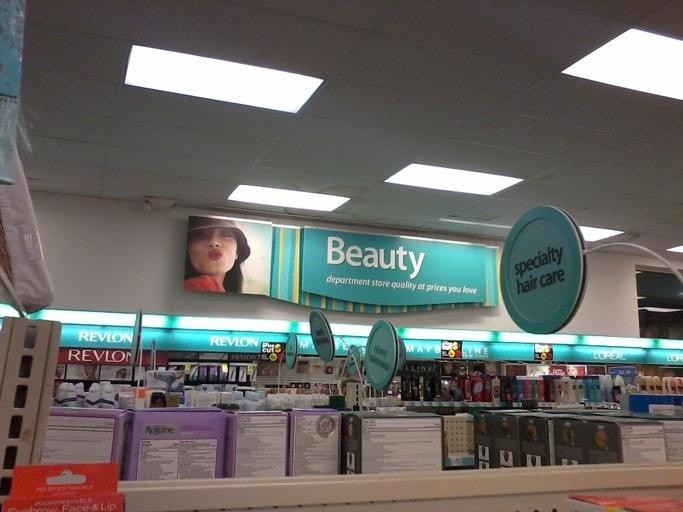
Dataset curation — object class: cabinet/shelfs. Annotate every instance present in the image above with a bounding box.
[120,467,543,512]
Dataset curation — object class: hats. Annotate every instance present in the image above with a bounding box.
[188,216,251,263]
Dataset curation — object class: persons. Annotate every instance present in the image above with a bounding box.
[151,392,169,411]
[186,217,250,296]
[55,362,65,379]
[82,363,99,382]
[442,363,454,374]
[486,361,498,377]
[114,367,127,380]
